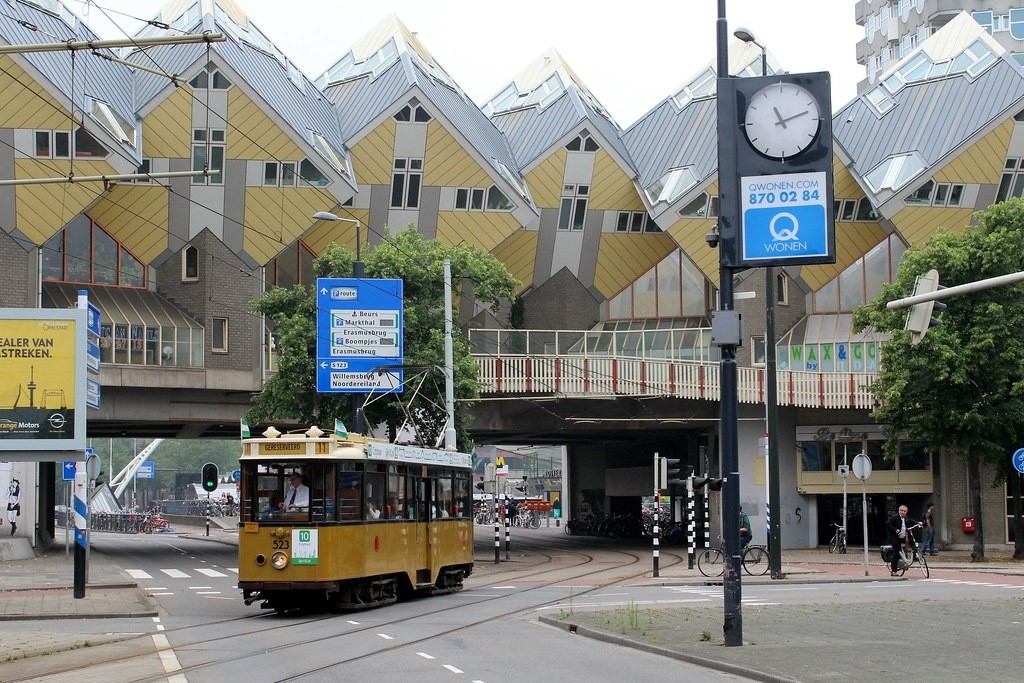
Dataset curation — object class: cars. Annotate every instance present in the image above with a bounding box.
[55,504,71,518]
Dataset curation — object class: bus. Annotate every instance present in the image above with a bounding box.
[238,426,475,618]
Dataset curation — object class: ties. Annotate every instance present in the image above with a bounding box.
[289,489,297,510]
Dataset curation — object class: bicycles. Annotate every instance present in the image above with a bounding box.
[210,500,240,517]
[880,522,929,578]
[828,523,847,555]
[139,518,169,533]
[476,507,541,529]
[564,512,689,543]
[697,536,771,576]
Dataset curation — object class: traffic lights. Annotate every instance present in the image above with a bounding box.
[667,458,680,488]
[478,483,484,492]
[205,466,215,489]
[710,478,722,491]
[929,285,949,330]
[95,471,104,488]
[693,477,711,488]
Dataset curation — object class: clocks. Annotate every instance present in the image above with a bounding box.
[741,81,825,164]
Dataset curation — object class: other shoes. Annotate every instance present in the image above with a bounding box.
[922,552,927,555]
[930,553,938,556]
[891,572,899,576]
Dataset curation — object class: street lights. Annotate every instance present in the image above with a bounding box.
[170,440,181,473]
[313,210,360,261]
[732,25,794,584]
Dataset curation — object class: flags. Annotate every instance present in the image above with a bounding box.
[241,418,250,438]
[335,418,348,439]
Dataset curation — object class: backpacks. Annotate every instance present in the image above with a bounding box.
[921,512,932,528]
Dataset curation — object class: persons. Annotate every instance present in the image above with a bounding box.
[380,496,449,519]
[278,472,310,512]
[739,504,752,565]
[498,498,523,526]
[219,492,234,517]
[887,505,923,576]
[921,502,937,556]
[365,497,380,520]
